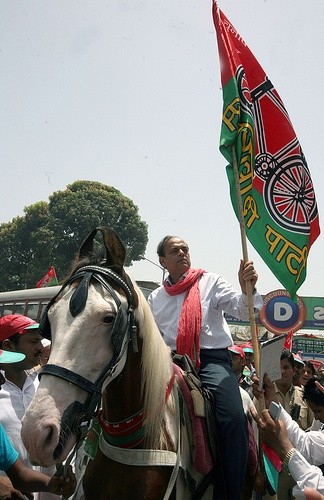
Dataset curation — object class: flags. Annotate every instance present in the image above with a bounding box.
[212,0,321,302]
[36,269,58,288]
[281,331,293,353]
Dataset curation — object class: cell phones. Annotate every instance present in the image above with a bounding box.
[268,401,281,421]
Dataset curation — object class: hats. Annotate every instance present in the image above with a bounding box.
[0,314,41,341]
[240,344,254,355]
[0,350,26,363]
[228,344,242,358]
[293,355,304,368]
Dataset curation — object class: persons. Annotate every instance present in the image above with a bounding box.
[227,341,324,500]
[146,236,245,500]
[0,313,78,500]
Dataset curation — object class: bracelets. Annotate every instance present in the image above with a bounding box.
[241,287,257,297]
[280,447,300,476]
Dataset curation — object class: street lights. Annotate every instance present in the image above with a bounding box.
[138,254,166,287]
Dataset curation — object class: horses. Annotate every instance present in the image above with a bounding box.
[22,227,260,500]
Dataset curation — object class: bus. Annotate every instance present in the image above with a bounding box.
[0,285,66,323]
